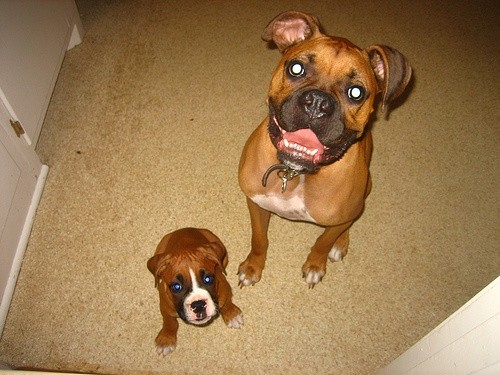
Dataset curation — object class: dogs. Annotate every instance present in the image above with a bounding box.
[236,9,412,291]
[147,227,246,357]
